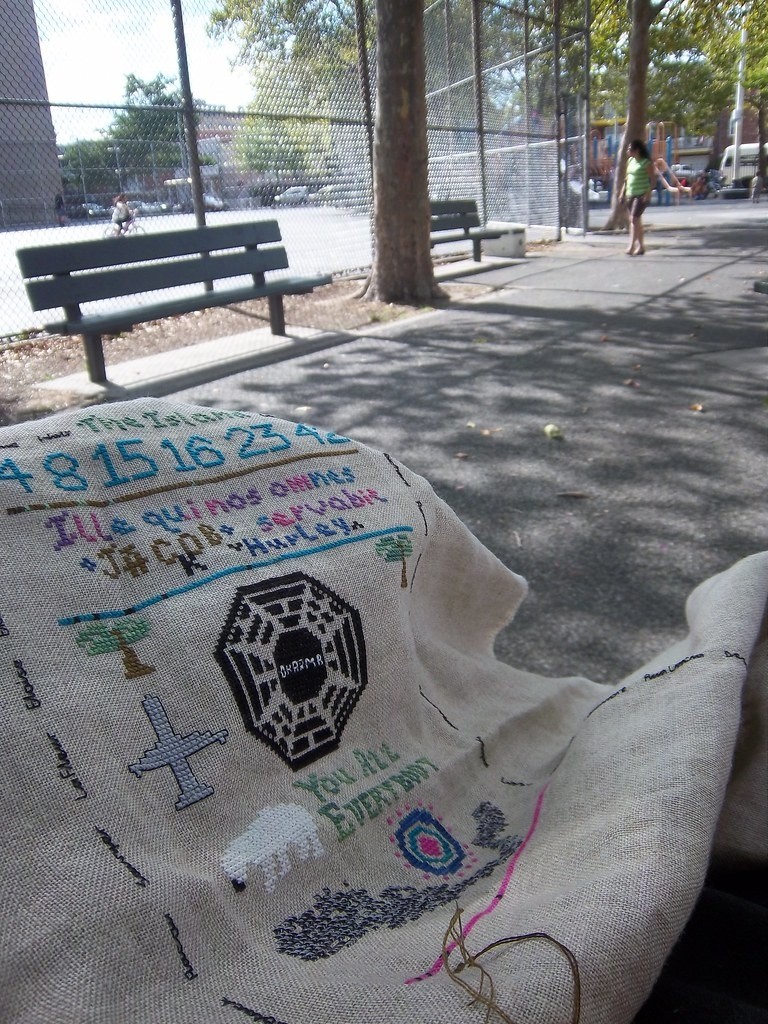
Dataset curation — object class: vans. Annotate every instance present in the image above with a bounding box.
[719,144,768,188]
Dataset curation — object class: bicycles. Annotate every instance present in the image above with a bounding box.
[102,212,147,238]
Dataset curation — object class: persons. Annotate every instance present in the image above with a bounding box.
[750,171,763,204]
[56,189,66,225]
[111,193,133,237]
[619,139,657,257]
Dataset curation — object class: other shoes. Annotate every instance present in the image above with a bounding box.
[625,246,645,256]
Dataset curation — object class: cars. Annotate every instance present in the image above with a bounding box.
[71,195,223,220]
[670,164,702,188]
[273,183,357,205]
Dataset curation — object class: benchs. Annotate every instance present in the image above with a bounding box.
[15,219,332,384]
[429,199,503,263]
